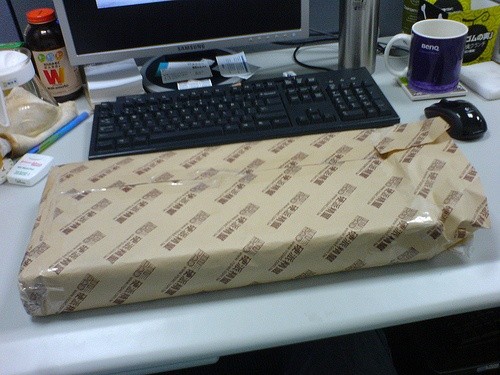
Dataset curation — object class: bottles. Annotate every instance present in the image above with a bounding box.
[24,7,81,104]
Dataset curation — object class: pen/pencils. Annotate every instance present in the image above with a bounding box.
[29,110,90,154]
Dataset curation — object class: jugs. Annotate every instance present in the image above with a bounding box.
[0,47,60,137]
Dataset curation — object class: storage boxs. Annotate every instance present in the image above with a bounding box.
[417,0,500,66]
[18,116,491,317]
[7,152,53,187]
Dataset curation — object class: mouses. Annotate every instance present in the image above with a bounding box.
[426,101,487,137]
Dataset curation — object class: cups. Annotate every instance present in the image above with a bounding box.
[384,19,470,90]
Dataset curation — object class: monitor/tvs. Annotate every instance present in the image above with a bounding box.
[50,0,310,93]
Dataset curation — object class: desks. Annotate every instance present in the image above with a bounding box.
[0,35,500,375]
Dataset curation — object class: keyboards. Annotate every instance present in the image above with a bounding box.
[88,67,400,160]
